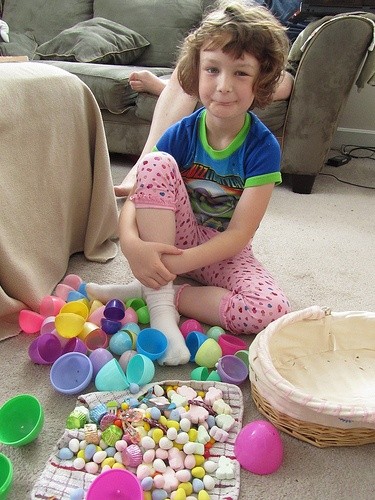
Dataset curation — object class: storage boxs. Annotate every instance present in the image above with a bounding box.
[248,304,375,448]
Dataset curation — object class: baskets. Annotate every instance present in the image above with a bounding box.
[247,305,375,448]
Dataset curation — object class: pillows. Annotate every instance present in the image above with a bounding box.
[34,17,150,65]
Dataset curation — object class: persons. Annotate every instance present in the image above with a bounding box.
[84,1,294,367]
[111,0,375,201]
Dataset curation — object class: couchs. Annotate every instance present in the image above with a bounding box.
[0,0,375,194]
